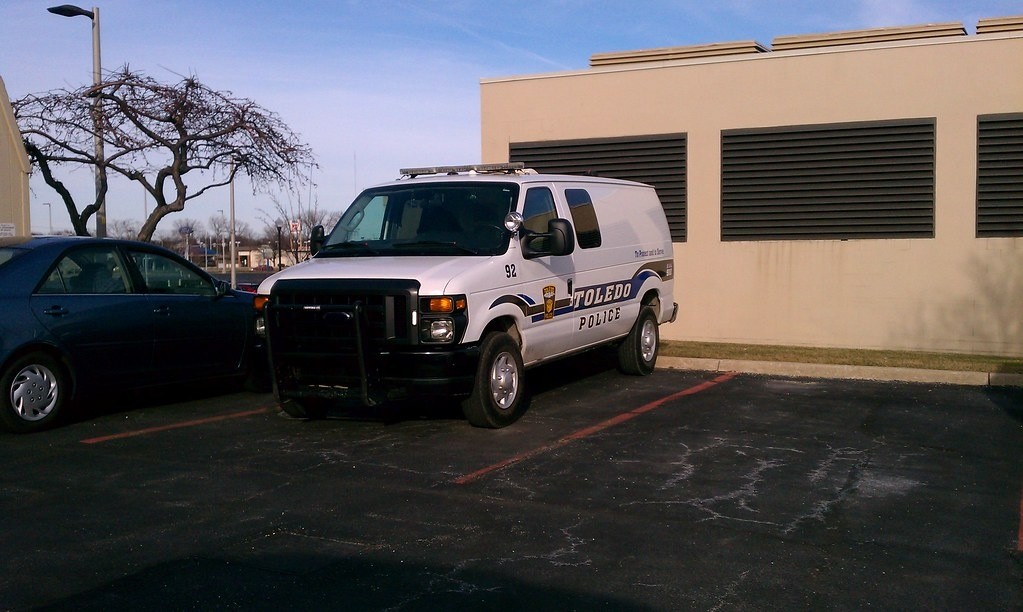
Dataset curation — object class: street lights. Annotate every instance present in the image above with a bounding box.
[275,216,284,272]
[47,4,107,236]
[43,202,52,236]
[216,209,227,273]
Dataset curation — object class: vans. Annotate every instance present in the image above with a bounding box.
[253,162,678,429]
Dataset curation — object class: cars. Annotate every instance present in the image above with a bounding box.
[0,236,256,432]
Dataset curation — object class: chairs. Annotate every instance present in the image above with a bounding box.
[74,264,126,294]
